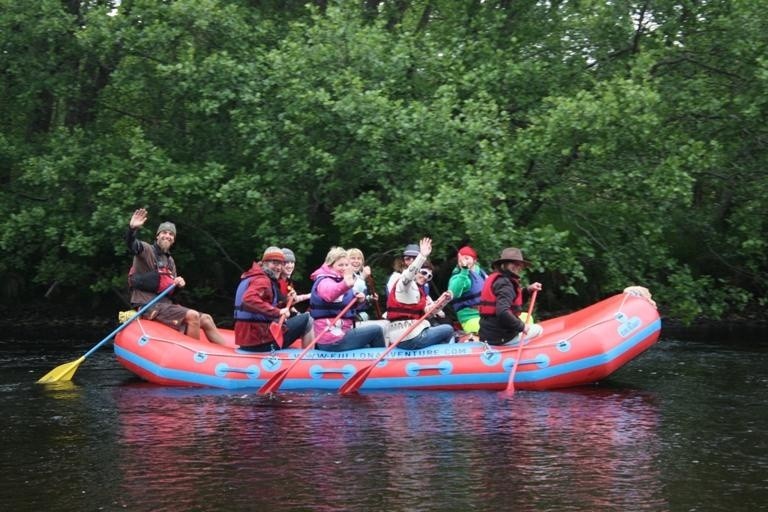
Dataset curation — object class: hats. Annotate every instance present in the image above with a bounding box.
[459,246,477,260]
[263,246,296,262]
[156,221,176,236]
[403,244,420,256]
[491,247,534,271]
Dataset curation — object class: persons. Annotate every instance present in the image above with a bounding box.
[277,247,312,316]
[386,235,454,351]
[386,243,446,325]
[477,246,544,346]
[447,246,534,336]
[344,247,379,321]
[231,246,316,353]
[128,207,232,347]
[309,246,387,352]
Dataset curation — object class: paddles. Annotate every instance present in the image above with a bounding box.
[504,290,538,397]
[337,294,448,395]
[256,292,359,393]
[268,292,295,348]
[36,283,176,382]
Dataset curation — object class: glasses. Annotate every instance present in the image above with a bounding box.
[420,268,433,281]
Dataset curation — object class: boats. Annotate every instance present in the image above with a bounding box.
[113,285,661,390]
[117,376,660,462]
